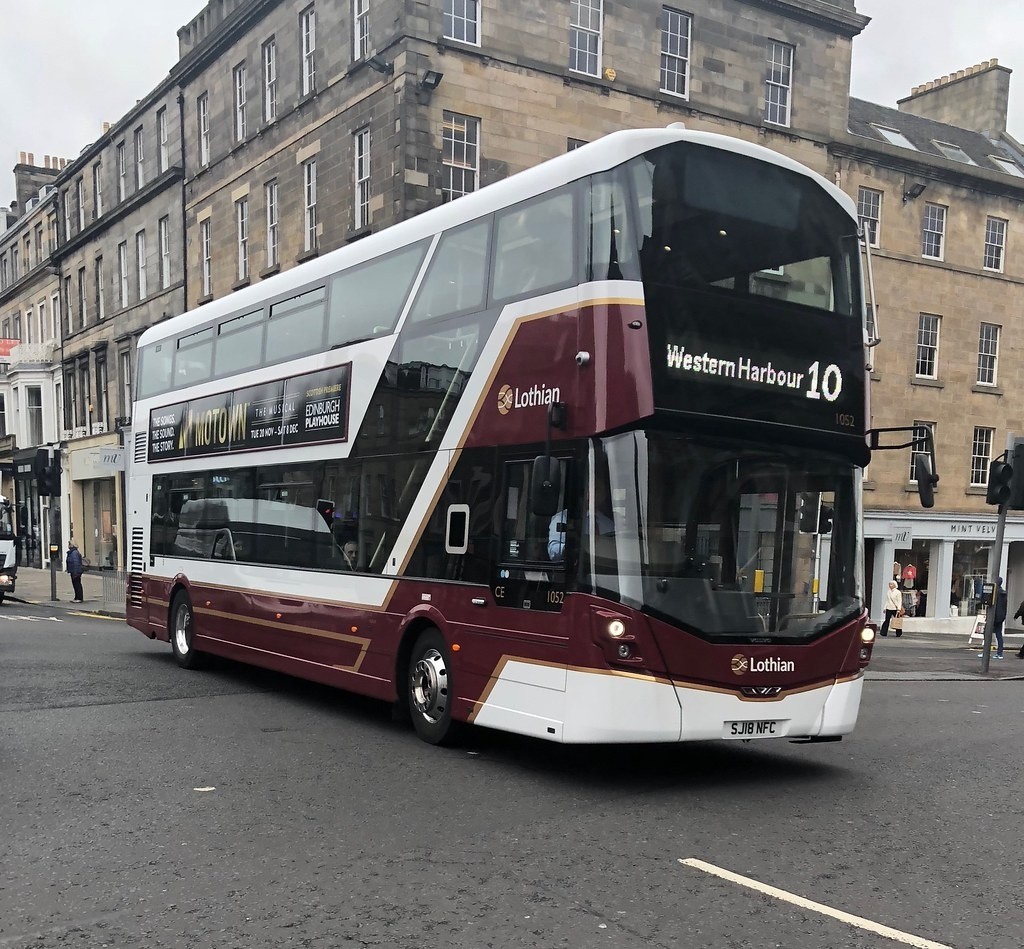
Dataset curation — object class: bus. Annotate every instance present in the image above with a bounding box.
[0,493,28,606]
[125,121,939,746]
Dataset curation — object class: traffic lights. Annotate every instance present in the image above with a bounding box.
[40,464,61,496]
[817,505,834,536]
[800,493,823,536]
[986,460,1013,506]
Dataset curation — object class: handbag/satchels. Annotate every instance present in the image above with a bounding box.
[890,612,902,630]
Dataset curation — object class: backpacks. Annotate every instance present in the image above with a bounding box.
[75,550,91,571]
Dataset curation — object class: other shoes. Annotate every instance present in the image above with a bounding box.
[992,654,1003,659]
[896,634,901,637]
[1015,653,1024,658]
[70,599,82,603]
[978,653,991,658]
[880,632,886,636]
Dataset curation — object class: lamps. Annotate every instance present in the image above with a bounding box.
[903,182,925,203]
[367,55,396,77]
[418,69,445,91]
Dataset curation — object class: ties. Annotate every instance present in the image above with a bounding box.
[588,516,600,536]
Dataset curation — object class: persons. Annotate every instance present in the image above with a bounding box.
[978,577,1007,659]
[547,478,615,562]
[879,580,903,638]
[1014,600,1024,658]
[66,540,85,603]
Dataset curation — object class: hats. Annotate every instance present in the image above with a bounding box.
[998,577,1002,585]
